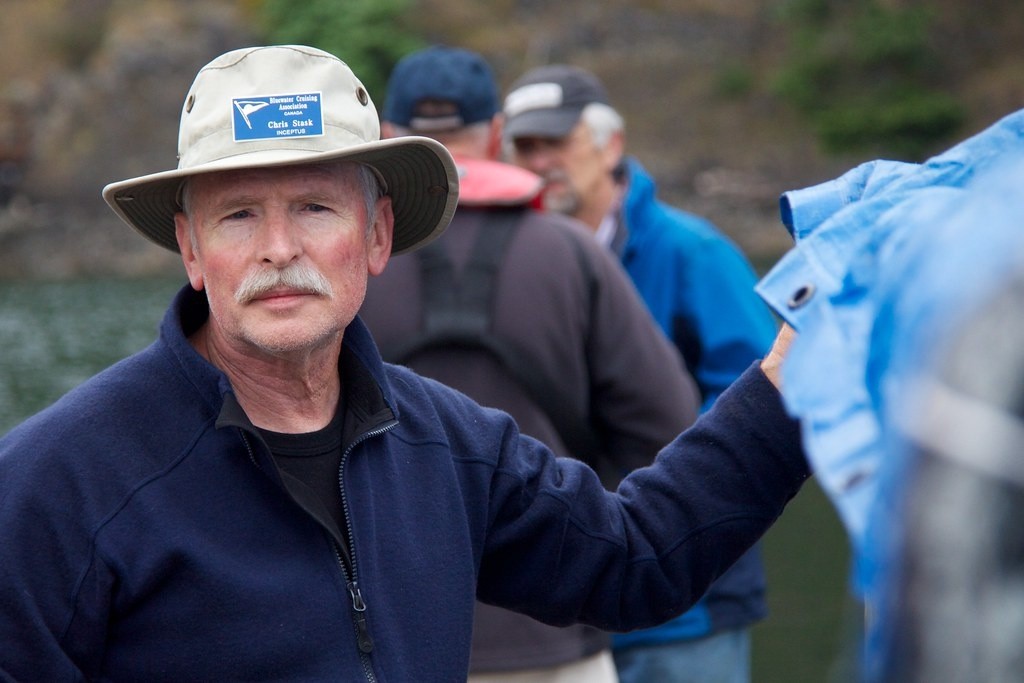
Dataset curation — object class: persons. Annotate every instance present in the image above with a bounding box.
[1,44,815,682]
[357,48,701,680]
[499,62,778,679]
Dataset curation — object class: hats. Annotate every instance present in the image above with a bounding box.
[100,44,458,261]
[495,63,614,141]
[377,52,501,134]
